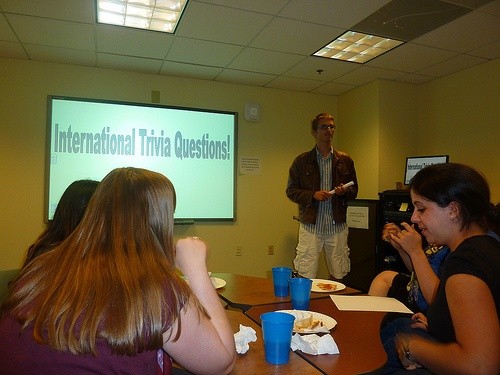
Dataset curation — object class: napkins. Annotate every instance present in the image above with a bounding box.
[290,332,339,355]
[233,324,257,355]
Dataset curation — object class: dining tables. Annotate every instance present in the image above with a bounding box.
[172,273,388,375]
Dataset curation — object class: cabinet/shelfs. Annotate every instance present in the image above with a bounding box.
[377,189,428,276]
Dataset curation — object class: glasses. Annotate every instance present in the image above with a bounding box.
[316,125,337,129]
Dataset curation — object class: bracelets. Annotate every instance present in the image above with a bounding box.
[404,332,412,361]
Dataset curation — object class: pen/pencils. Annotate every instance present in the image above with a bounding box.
[416,318,428,327]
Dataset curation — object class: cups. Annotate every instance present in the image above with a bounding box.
[288,278,313,310]
[260,313,295,365]
[272,268,292,296]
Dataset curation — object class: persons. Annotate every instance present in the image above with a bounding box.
[0,167,237,375]
[9,179,101,284]
[395,163,500,375]
[285,114,359,282]
[368,204,500,312]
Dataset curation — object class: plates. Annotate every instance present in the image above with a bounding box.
[275,310,337,333]
[182,277,226,289]
[310,279,346,292]
